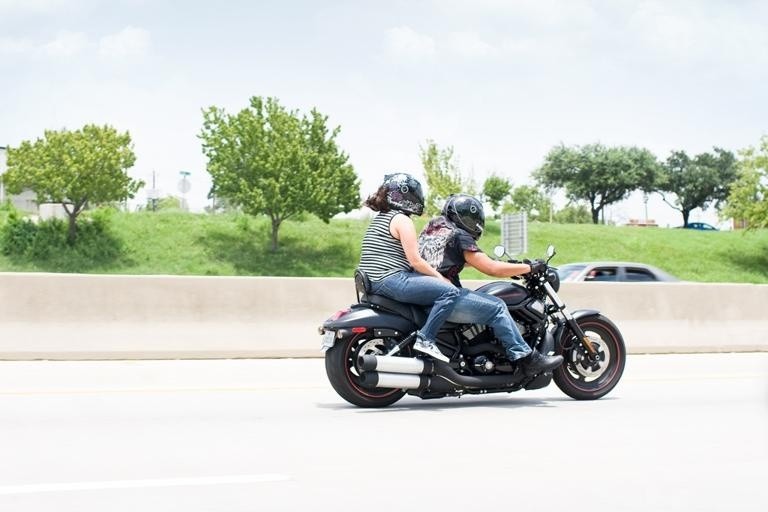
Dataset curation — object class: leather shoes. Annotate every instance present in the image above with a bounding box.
[519,346,565,377]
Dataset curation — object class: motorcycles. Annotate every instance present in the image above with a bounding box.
[318,245,626,407]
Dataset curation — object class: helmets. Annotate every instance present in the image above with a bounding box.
[380,172,425,217]
[441,194,485,241]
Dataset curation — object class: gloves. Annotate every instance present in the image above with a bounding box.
[527,258,549,276]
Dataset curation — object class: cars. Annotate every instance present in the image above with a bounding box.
[552,260,675,282]
[676,222,718,230]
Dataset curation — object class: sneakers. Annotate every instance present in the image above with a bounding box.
[411,336,450,364]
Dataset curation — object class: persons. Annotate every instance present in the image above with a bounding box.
[360,173,461,362]
[411,192,564,376]
